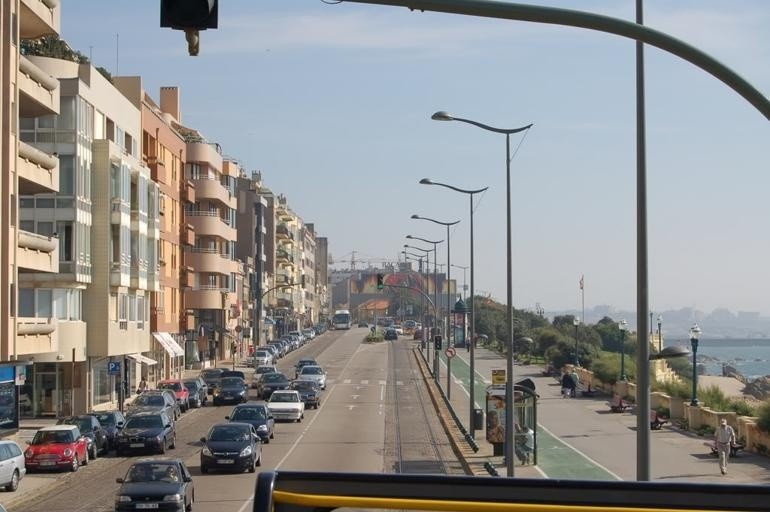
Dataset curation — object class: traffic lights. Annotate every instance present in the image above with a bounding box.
[377,272,384,290]
[434,335,442,351]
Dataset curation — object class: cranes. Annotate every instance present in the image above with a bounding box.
[328,248,367,270]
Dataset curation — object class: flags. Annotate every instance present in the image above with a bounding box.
[580,277,583,290]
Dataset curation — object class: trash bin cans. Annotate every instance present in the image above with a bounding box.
[473,409,483,430]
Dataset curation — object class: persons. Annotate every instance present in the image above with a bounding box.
[714,419,736,475]
[139,376,149,392]
[562,371,579,399]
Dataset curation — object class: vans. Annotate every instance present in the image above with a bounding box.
[0,440,26,491]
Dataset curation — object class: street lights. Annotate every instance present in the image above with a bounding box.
[657,315,663,360]
[573,315,580,366]
[160,2,769,115]
[420,177,489,441]
[410,214,461,401]
[401,244,469,363]
[688,323,702,406]
[617,318,628,380]
[649,308,654,334]
[430,111,533,480]
[405,234,444,378]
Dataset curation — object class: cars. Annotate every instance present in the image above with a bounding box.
[19,388,32,411]
[200,422,263,473]
[224,403,274,444]
[125,317,334,422]
[358,314,459,341]
[88,409,126,444]
[117,410,176,455]
[115,456,194,511]
[55,414,109,460]
[23,424,92,474]
[0,393,15,418]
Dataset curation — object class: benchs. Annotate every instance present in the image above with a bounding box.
[702,440,718,454]
[730,441,744,457]
[648,408,656,431]
[656,415,668,429]
[581,384,630,417]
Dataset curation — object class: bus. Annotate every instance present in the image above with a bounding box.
[333,309,352,330]
